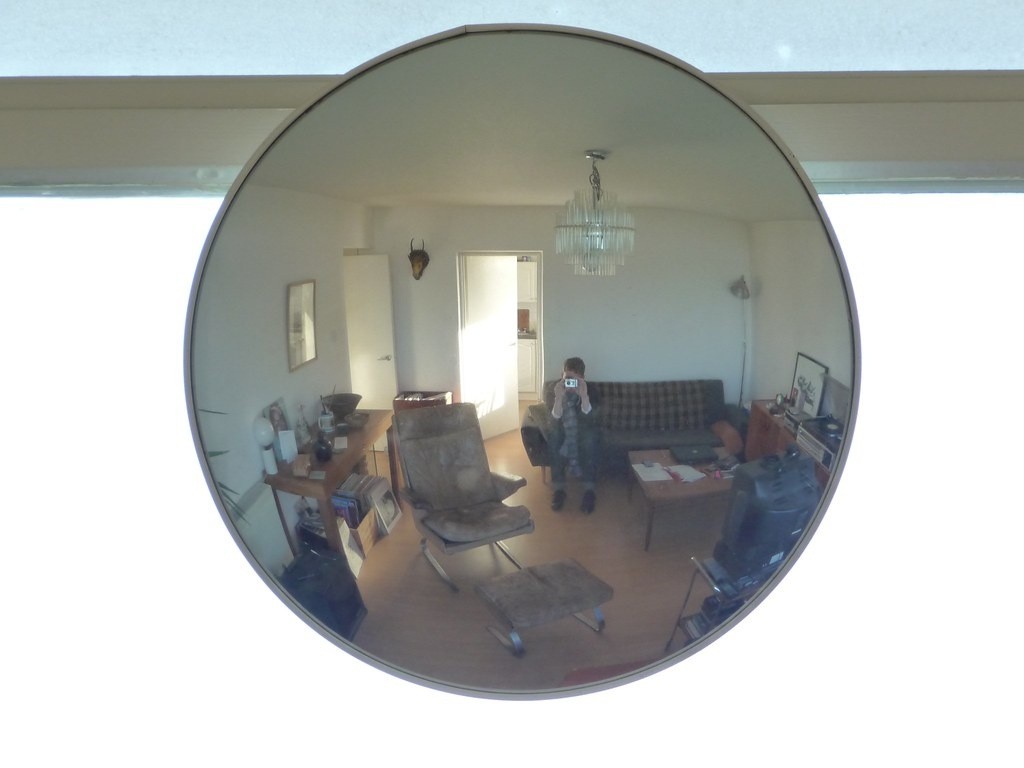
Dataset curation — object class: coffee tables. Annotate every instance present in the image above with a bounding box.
[628,446,742,552]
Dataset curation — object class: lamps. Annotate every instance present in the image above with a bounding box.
[553,149,635,276]
[253,417,278,475]
[730,276,750,430]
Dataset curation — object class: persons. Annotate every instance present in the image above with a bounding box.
[545,358,600,513]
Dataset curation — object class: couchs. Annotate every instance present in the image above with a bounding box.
[521,380,743,484]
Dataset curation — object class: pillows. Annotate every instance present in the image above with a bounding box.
[529,403,553,442]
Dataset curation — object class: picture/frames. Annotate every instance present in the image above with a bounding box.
[789,352,829,418]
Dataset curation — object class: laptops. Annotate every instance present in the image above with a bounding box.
[670,444,719,464]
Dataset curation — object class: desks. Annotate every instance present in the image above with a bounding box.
[265,409,404,554]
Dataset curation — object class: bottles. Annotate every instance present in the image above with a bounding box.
[314,430,332,462]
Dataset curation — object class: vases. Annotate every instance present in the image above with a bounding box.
[324,393,362,419]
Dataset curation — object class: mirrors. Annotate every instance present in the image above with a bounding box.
[183,24,862,703]
[287,279,317,372]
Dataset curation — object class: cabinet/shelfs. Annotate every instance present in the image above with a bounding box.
[663,556,765,657]
[518,339,538,401]
[744,399,830,488]
[517,262,537,303]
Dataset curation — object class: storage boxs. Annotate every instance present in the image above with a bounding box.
[349,508,379,559]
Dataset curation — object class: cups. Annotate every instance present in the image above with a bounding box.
[318,411,334,432]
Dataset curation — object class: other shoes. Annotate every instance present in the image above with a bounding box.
[580,489,595,514]
[550,490,564,510]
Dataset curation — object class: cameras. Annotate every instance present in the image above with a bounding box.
[564,378,579,388]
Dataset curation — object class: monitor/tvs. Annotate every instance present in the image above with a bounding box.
[722,447,821,564]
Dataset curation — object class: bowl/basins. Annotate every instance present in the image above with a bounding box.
[322,394,362,420]
[344,413,369,429]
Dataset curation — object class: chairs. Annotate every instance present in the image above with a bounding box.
[392,402,614,658]
